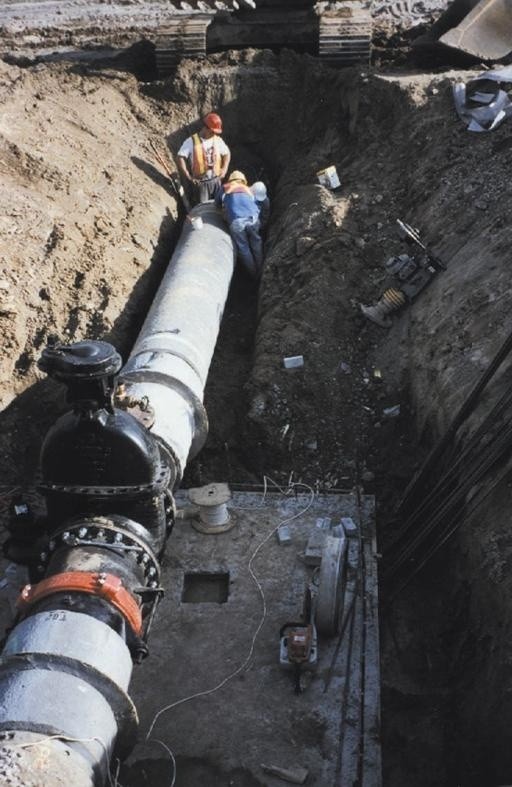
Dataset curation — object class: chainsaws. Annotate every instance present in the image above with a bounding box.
[280,583,318,696]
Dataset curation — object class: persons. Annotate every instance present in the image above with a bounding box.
[250,181,271,242]
[214,169,264,275]
[177,113,232,206]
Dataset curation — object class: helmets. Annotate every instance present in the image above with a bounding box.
[228,171,247,184]
[252,181,268,201]
[204,114,222,133]
